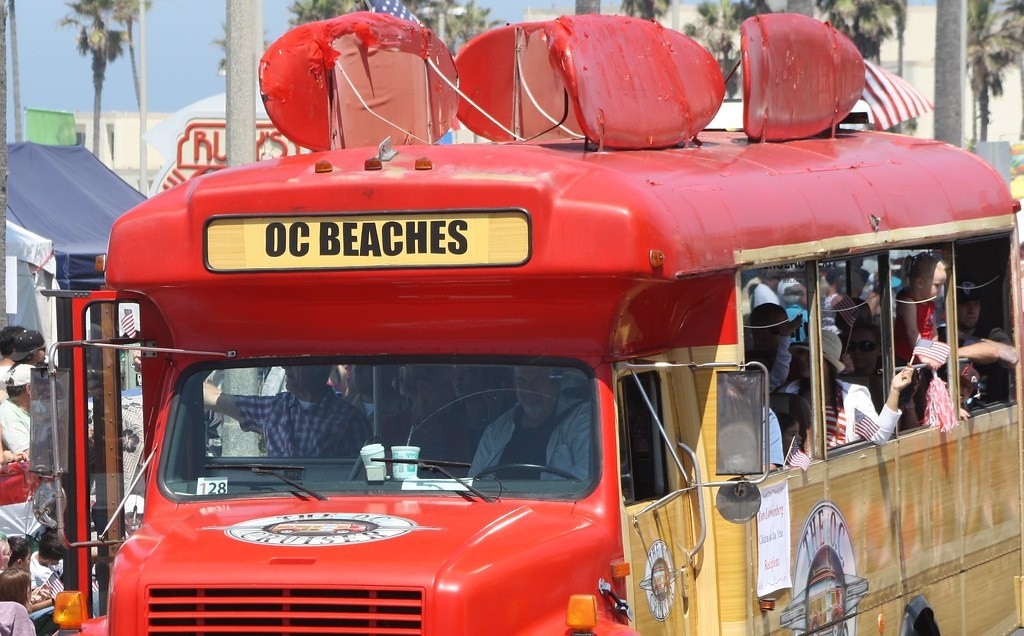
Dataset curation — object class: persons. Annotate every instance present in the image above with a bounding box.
[741,251,1020,475]
[0,326,145,636]
[133,350,590,494]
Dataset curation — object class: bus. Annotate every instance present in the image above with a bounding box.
[30,14,1024,636]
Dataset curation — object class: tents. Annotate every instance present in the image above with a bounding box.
[6,141,148,356]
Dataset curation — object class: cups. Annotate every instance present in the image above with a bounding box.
[360,444,386,476]
[392,447,420,481]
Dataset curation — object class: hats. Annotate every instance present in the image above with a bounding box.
[957,361,980,390]
[123,494,145,516]
[956,276,987,304]
[10,329,45,362]
[3,362,39,387]
[788,328,845,374]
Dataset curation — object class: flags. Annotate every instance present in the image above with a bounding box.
[854,407,880,442]
[120,308,137,339]
[912,338,951,365]
[786,436,810,472]
[44,572,64,600]
[831,292,859,327]
[364,0,460,131]
[859,59,934,132]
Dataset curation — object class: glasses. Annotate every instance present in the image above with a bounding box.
[845,340,881,353]
